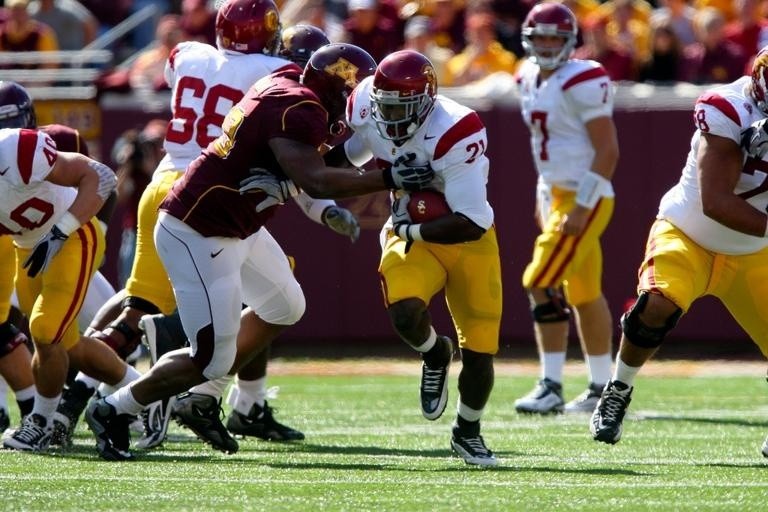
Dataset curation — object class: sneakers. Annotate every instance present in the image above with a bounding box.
[3,413,54,453]
[564,388,600,413]
[450,433,499,468]
[49,419,74,451]
[419,336,454,421]
[760,434,767,458]
[85,395,138,461]
[589,378,634,444]
[137,311,187,369]
[134,395,176,450]
[171,389,239,454]
[226,398,306,441]
[513,376,564,414]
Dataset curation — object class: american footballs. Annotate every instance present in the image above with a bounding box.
[405,187,452,224]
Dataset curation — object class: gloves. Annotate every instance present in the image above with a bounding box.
[22,224,70,278]
[740,118,767,161]
[239,166,292,214]
[390,189,412,236]
[324,206,361,244]
[382,152,435,191]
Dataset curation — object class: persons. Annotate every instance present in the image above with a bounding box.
[0,0,768,466]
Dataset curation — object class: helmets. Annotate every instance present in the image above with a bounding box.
[368,49,438,142]
[282,24,330,65]
[299,41,379,116]
[749,44,768,115]
[214,0,281,54]
[0,80,37,129]
[521,1,578,71]
